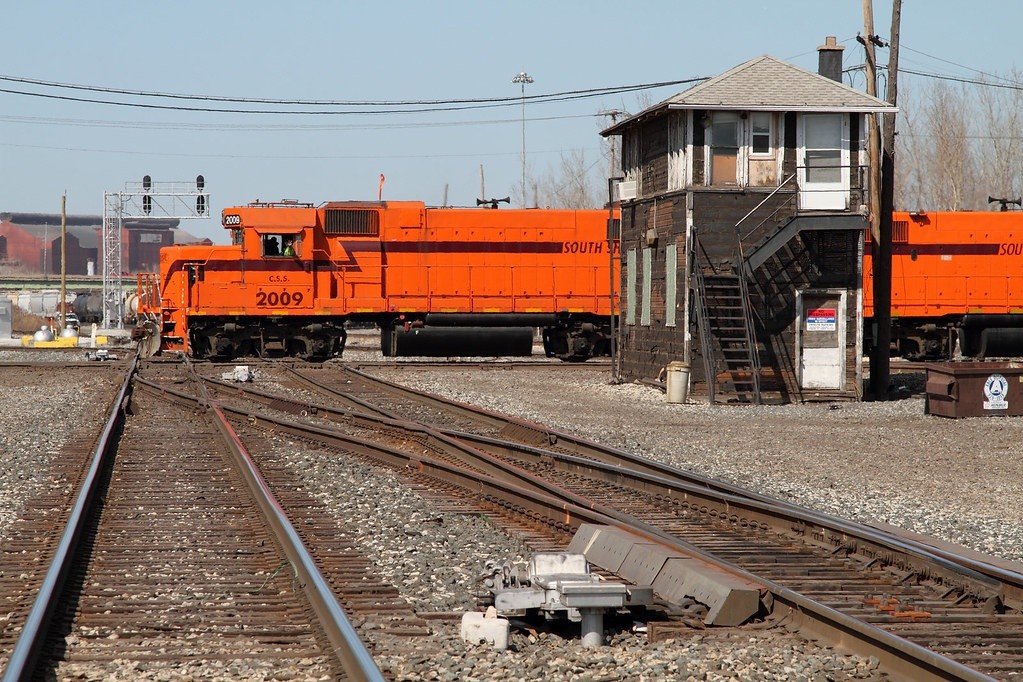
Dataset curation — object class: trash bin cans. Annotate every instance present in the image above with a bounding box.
[666,360,692,403]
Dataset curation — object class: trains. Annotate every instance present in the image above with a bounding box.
[123,198,1022,365]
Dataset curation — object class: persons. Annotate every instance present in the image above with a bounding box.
[283,239,294,255]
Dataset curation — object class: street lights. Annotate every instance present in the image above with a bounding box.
[510,71,535,207]
